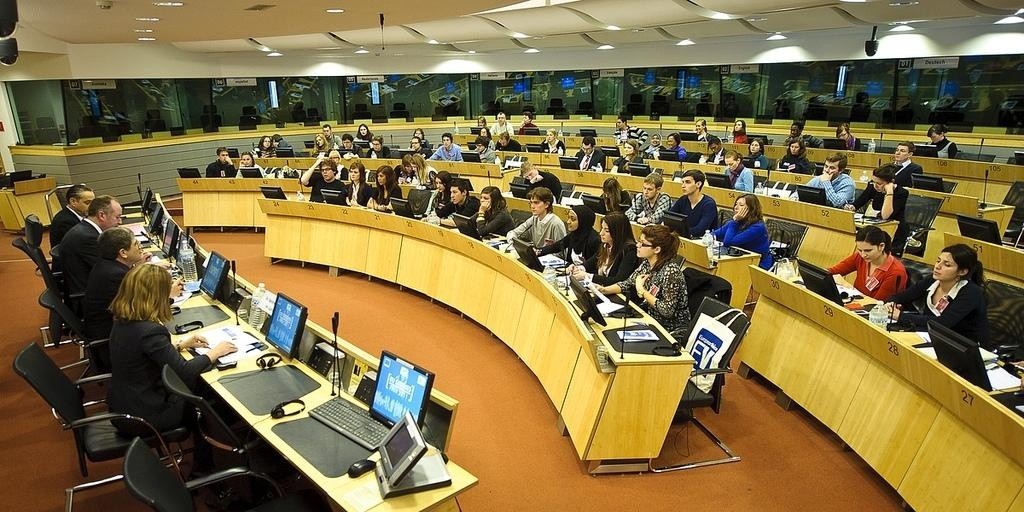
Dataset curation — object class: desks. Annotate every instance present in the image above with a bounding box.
[0,177,63,234]
[118,194,480,512]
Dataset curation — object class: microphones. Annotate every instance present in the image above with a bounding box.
[620,292,631,359]
[777,230,786,276]
[232,261,241,326]
[560,241,570,299]
[890,276,902,335]
[719,213,725,258]
[331,317,345,398]
[331,312,340,397]
[505,225,533,254]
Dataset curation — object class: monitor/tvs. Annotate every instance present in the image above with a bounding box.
[928,320,993,393]
[201,251,231,305]
[141,186,196,274]
[370,350,436,434]
[512,237,546,273]
[797,259,845,308]
[569,275,608,328]
[266,292,309,362]
[9,169,33,188]
[176,120,1007,247]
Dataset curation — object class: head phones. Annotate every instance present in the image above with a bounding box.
[271,399,306,419]
[653,343,683,357]
[257,354,282,370]
[175,321,204,335]
[841,292,855,305]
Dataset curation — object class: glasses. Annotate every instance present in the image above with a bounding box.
[320,168,332,173]
[636,238,656,248]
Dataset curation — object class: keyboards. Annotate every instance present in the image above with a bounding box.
[309,396,392,452]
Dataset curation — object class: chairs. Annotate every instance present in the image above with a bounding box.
[39,288,109,379]
[161,363,297,460]
[123,435,332,511]
[31,248,86,347]
[48,244,60,275]
[25,215,53,277]
[11,339,203,511]
[12,238,63,288]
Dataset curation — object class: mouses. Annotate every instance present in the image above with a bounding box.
[348,459,377,478]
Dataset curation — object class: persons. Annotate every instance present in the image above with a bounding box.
[107,263,238,482]
[83,225,183,371]
[924,123,960,163]
[59,194,173,324]
[47,185,95,274]
[205,113,921,342]
[886,241,988,351]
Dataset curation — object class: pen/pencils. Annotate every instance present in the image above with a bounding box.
[246,344,264,353]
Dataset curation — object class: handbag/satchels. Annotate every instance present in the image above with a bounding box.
[762,186,791,201]
[561,196,583,209]
[773,258,800,279]
[504,159,525,168]
[684,313,737,395]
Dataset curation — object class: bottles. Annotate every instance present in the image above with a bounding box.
[702,229,714,260]
[868,137,877,151]
[495,155,501,166]
[728,130,734,142]
[754,182,764,193]
[558,147,563,155]
[296,190,304,201]
[371,151,378,158]
[543,259,556,284]
[178,240,198,282]
[430,208,439,224]
[249,282,267,330]
[596,163,603,172]
[869,300,890,328]
[860,169,869,179]
[557,130,563,136]
